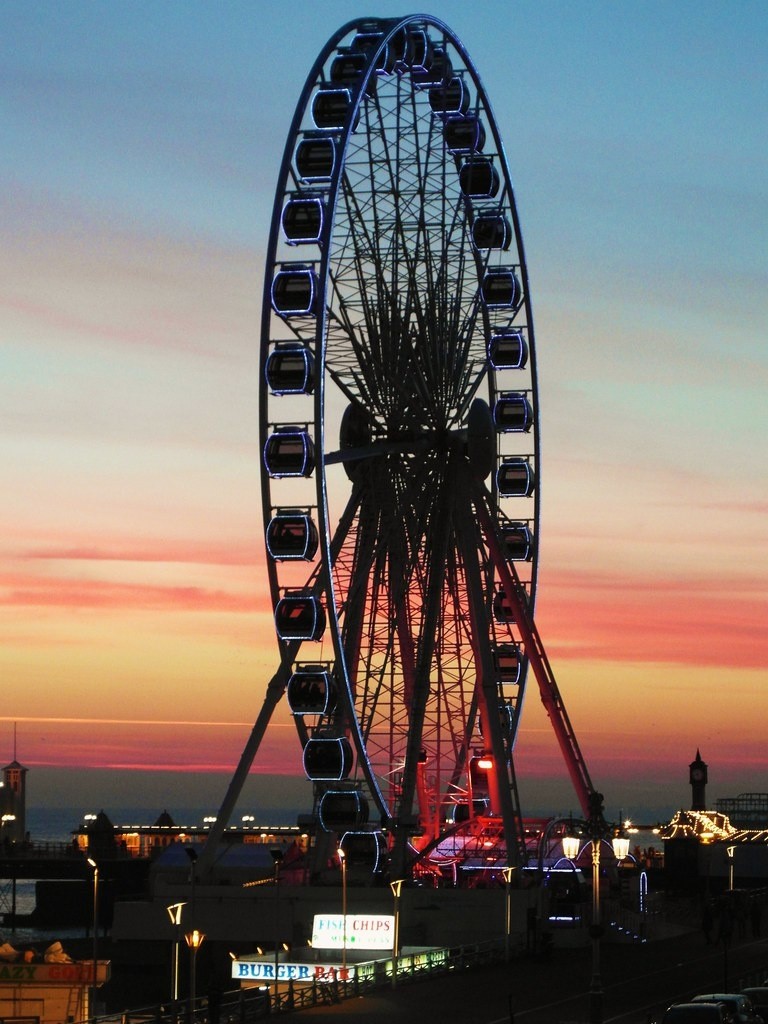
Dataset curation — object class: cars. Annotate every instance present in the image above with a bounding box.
[660,980,768,1024]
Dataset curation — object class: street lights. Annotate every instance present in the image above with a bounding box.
[338,848,349,998]
[560,807,633,1024]
[391,880,407,988]
[184,930,207,1024]
[726,844,739,892]
[86,857,98,1024]
[501,866,518,963]
[166,900,192,1024]
[268,848,288,1011]
